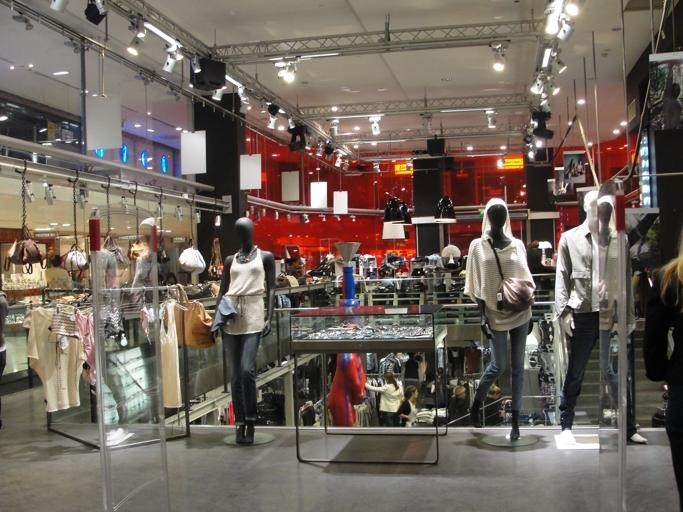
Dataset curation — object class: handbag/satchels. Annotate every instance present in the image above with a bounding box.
[64,243,91,272]
[102,235,130,271]
[6,238,43,266]
[497,276,536,313]
[163,282,219,350]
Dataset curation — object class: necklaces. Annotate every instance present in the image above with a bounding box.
[237,244,257,262]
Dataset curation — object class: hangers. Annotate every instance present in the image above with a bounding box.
[25,281,187,327]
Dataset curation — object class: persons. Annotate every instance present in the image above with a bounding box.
[285,257,302,279]
[429,360,453,404]
[364,372,404,424]
[483,382,507,425]
[177,247,206,283]
[464,198,536,440]
[375,256,397,277]
[627,331,647,445]
[599,194,636,407]
[211,216,276,444]
[531,239,542,272]
[0,275,10,426]
[554,191,599,437]
[125,215,155,296]
[641,205,681,507]
[43,254,72,298]
[661,84,681,131]
[525,242,538,274]
[410,256,429,293]
[565,158,580,178]
[300,399,316,428]
[576,159,584,175]
[448,385,470,426]
[397,384,419,427]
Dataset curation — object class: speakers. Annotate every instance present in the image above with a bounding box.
[427,139,445,156]
[190,59,226,90]
